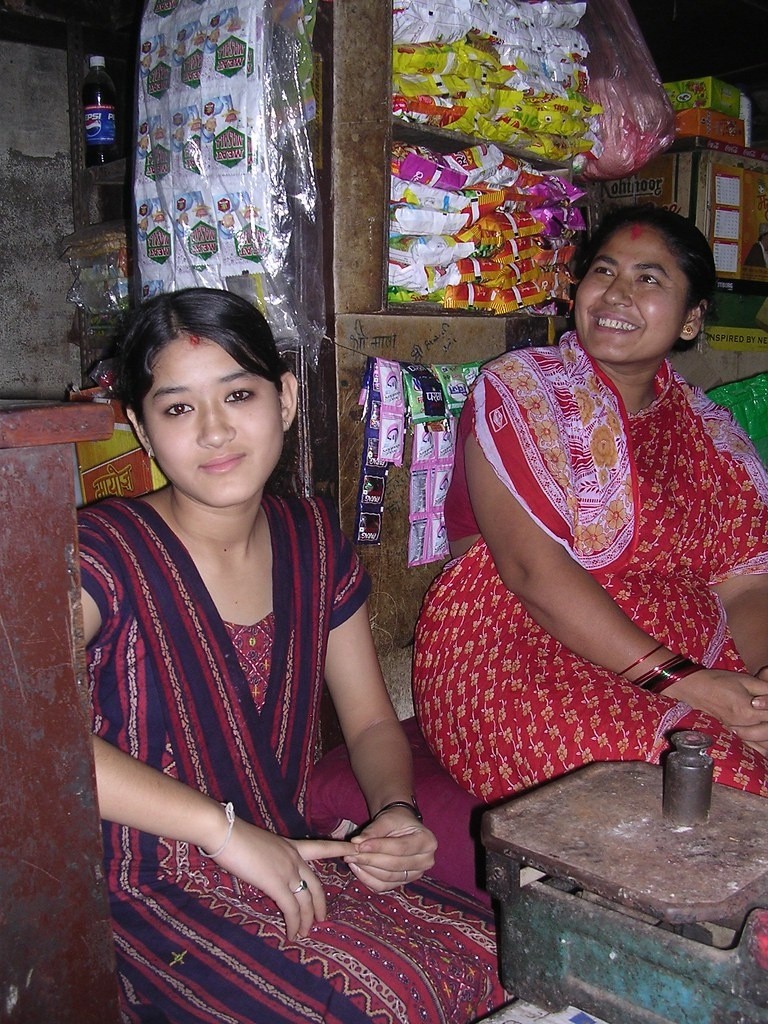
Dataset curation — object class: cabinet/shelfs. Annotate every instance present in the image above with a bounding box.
[63,0,584,386]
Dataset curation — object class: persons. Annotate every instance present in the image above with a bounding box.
[62,288,507,1024]
[413,203,768,805]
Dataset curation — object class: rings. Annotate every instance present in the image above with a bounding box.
[293,880,307,894]
[404,871,408,881]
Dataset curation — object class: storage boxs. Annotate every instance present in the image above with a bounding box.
[597,76,768,291]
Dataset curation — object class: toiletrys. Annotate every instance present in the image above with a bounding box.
[348,353,480,568]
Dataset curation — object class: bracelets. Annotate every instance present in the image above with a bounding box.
[197,803,235,858]
[620,644,664,675]
[373,796,423,823]
[631,654,705,694]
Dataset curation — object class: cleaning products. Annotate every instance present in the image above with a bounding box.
[386,0,604,316]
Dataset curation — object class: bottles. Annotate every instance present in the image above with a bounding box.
[82,56,120,167]
